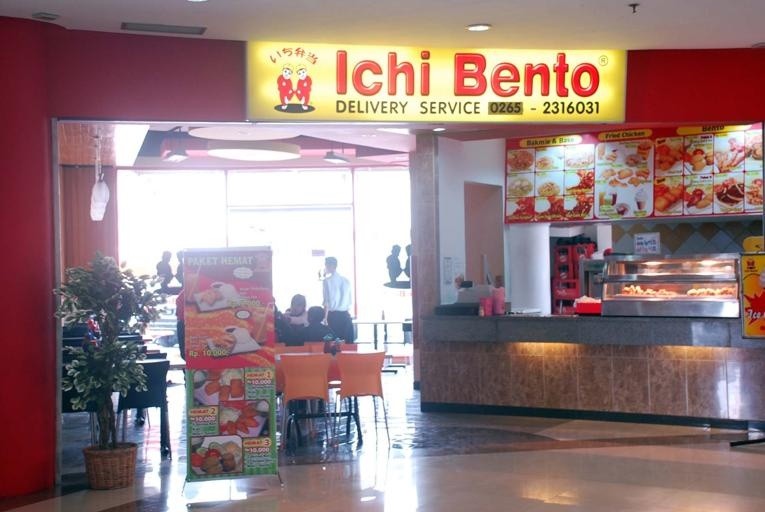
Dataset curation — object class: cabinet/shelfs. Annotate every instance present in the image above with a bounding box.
[596,252,742,320]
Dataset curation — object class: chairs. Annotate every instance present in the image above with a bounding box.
[276,339,390,457]
[64,335,178,462]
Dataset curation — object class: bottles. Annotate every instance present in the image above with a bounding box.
[478,296,494,318]
[492,286,505,316]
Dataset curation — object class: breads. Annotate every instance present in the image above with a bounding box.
[687,287,739,296]
[621,284,667,295]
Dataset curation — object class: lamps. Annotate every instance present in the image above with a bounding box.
[164,125,189,164]
[88,125,111,223]
[323,141,349,167]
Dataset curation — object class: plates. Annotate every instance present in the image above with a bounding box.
[193,289,241,311]
[206,334,262,357]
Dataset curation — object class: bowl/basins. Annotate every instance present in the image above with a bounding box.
[210,282,225,292]
[223,326,238,335]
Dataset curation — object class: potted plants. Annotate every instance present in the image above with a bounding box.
[53,250,155,491]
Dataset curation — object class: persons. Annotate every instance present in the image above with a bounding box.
[173,264,187,364]
[283,294,309,326]
[291,305,338,416]
[317,255,356,344]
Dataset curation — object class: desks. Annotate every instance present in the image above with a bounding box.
[350,317,406,373]
